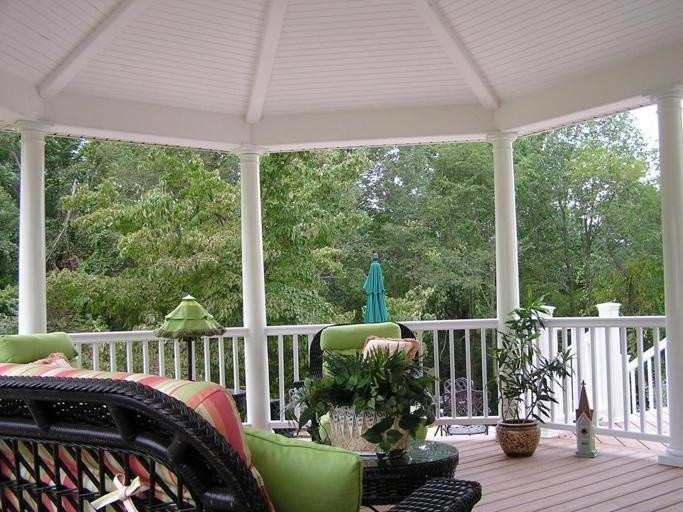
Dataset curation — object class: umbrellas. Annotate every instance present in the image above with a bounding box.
[361,253,388,323]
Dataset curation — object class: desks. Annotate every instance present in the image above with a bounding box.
[226,387,247,423]
[430,394,466,436]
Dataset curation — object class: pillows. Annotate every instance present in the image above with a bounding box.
[0,332,79,364]
[242,425,363,512]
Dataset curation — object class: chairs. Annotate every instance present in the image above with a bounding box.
[0,377,482,511]
[309,322,420,444]
[441,390,490,436]
[444,379,475,407]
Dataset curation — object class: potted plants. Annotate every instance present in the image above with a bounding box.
[486,285,577,457]
[290,348,435,453]
